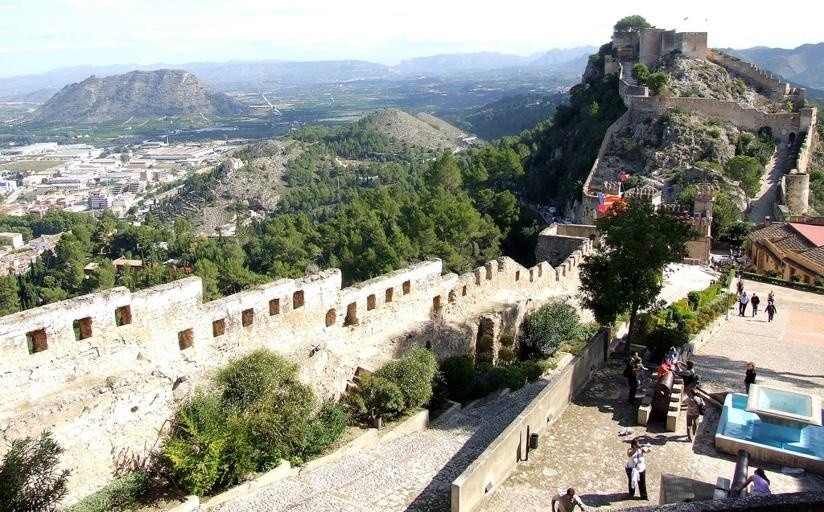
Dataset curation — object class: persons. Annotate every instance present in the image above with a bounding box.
[621,242,779,441]
[616,171,642,192]
[550,488,588,512]
[734,465,771,496]
[624,439,653,501]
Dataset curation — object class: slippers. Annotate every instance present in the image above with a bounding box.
[692,396,707,416]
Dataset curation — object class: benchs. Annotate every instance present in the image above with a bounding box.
[597,192,620,205]
[596,204,612,217]
